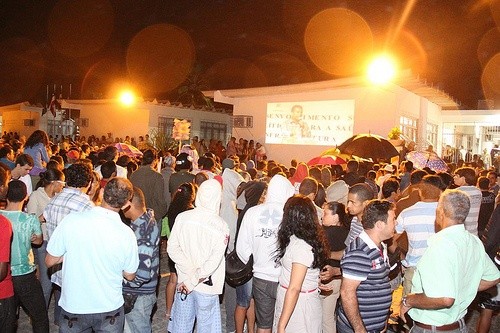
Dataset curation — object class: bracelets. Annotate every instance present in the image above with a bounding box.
[399,294,412,308]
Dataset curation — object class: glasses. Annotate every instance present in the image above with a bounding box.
[50,180,66,186]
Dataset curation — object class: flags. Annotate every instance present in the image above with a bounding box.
[41,92,61,117]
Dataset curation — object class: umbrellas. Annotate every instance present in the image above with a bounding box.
[319,146,364,163]
[404,148,449,172]
[337,128,398,158]
[306,154,347,166]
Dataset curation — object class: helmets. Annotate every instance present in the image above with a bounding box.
[225,249,253,288]
[175,152,193,172]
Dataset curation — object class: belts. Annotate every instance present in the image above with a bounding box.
[280,285,317,293]
[414,320,463,331]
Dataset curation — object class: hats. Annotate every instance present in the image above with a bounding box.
[222,158,238,170]
[66,150,79,159]
[382,165,395,171]
[246,160,256,170]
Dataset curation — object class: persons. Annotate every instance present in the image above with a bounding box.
[281,104,311,137]
[0,132,500,333]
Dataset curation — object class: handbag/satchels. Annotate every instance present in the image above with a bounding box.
[123,294,138,314]
[388,247,401,280]
